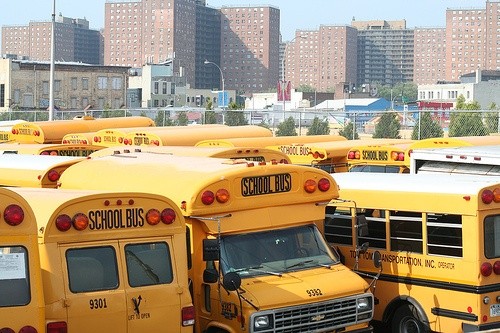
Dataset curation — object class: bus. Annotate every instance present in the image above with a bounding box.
[0,185,196,333]
[0,115,500,179]
[56,153,383,333]
[326,171,500,333]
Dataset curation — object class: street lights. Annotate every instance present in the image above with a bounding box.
[33,63,40,121]
[163,105,174,127]
[282,35,307,122]
[392,93,402,112]
[204,59,225,126]
